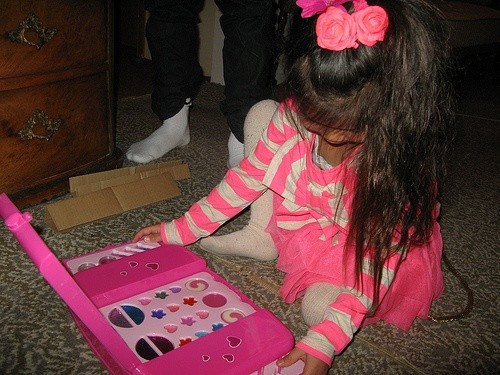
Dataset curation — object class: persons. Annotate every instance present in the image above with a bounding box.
[134,0,447,375]
[124,0,282,172]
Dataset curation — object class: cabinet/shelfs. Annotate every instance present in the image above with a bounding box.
[0,0,124,212]
